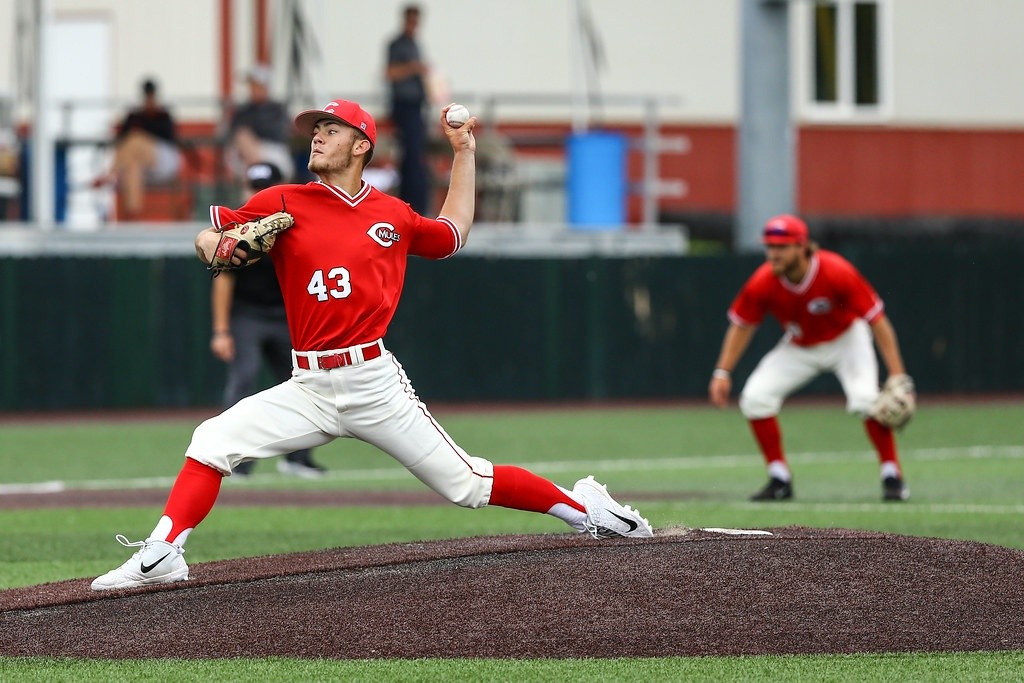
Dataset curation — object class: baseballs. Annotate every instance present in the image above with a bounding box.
[445,105,471,128]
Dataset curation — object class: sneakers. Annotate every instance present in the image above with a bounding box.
[91,535,189,590]
[572,475,654,540]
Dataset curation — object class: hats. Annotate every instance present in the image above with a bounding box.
[245,163,284,189]
[761,215,809,246]
[294,98,377,146]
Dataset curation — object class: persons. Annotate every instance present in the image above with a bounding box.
[385,8,433,216]
[710,215,914,502]
[211,162,330,480]
[93,81,180,220]
[215,68,294,207]
[92,100,652,592]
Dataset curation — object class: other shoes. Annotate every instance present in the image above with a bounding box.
[278,449,329,479]
[750,477,793,502]
[881,475,910,501]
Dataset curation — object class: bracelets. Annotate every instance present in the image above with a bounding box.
[214,329,231,335]
[712,368,731,377]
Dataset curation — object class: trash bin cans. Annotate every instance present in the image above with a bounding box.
[563,134,627,230]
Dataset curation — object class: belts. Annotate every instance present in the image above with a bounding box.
[296,343,381,370]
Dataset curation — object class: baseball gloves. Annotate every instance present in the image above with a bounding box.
[206,193,295,281]
[866,374,917,435]
